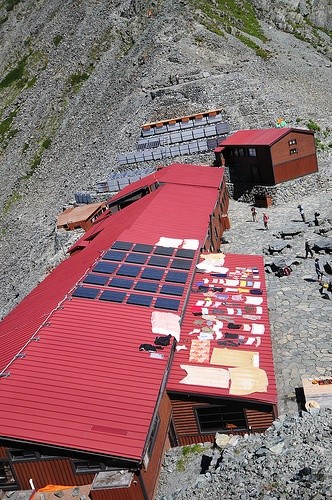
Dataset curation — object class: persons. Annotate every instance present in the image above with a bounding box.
[298,205,305,222]
[263,212,269,229]
[315,259,324,281]
[305,242,314,258]
[251,206,256,222]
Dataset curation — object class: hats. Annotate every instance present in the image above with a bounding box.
[297,205,302,208]
[315,258,319,262]
[305,238,309,242]
